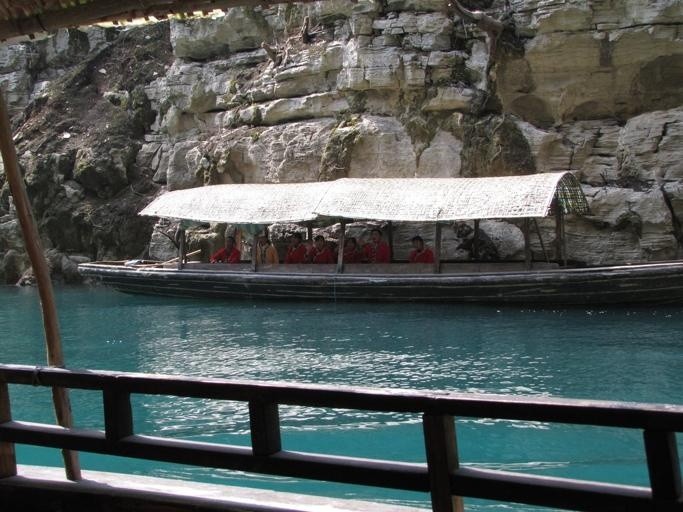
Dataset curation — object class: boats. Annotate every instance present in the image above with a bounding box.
[75,171,683,307]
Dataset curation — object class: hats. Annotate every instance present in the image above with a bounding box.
[314,235,325,240]
[289,233,302,240]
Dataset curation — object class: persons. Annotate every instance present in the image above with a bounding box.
[254,233,279,264]
[310,235,334,263]
[362,229,390,263]
[408,236,433,264]
[339,238,361,264]
[282,233,307,264]
[207,235,240,264]
